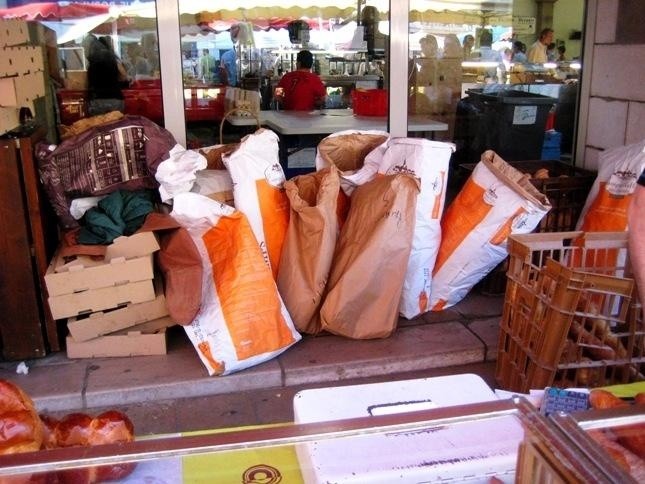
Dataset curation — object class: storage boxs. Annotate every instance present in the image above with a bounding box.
[291,372,526,483]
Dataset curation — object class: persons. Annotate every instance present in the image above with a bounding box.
[218,21,266,88]
[556,45,567,61]
[509,41,527,63]
[626,168,645,312]
[271,21,322,84]
[416,31,507,114]
[85,39,128,116]
[276,50,326,111]
[349,4,390,75]
[121,41,149,75]
[191,22,221,65]
[527,27,553,63]
[547,41,556,61]
[200,48,216,83]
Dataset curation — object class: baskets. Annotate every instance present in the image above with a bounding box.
[496,232,645,395]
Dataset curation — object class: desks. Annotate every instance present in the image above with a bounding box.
[226,109,449,174]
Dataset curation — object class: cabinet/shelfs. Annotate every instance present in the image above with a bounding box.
[0,106,66,367]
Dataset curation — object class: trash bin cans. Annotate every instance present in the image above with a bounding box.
[465,88,559,161]
